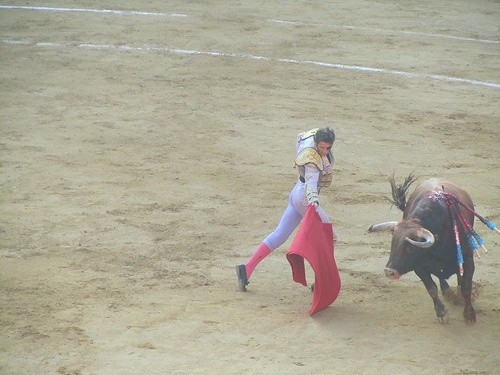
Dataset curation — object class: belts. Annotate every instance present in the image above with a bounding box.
[300,176,305,183]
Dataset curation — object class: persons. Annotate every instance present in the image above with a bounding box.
[235,126,336,293]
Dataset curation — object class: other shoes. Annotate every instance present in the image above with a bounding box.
[235,264,250,292]
[311,283,315,291]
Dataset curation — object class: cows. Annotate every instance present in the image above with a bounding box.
[367,171,478,327]
